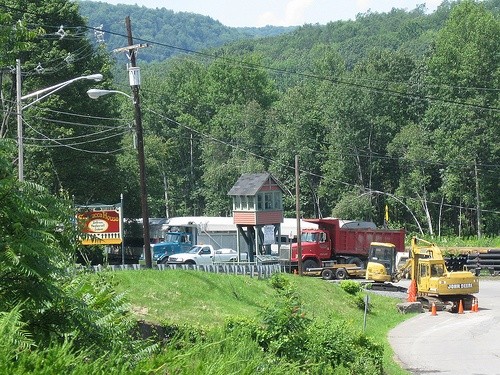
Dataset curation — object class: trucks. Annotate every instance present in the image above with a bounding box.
[289,218,406,281]
[140,221,253,266]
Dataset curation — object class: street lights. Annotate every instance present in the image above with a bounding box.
[86,88,153,269]
[15,58,104,184]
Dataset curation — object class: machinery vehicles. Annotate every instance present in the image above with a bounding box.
[365,241,413,291]
[410,236,481,314]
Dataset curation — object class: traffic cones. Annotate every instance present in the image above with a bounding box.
[475,298,479,312]
[456,299,466,314]
[430,303,438,316]
[470,297,475,312]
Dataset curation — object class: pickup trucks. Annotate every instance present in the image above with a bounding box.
[168,244,248,263]
